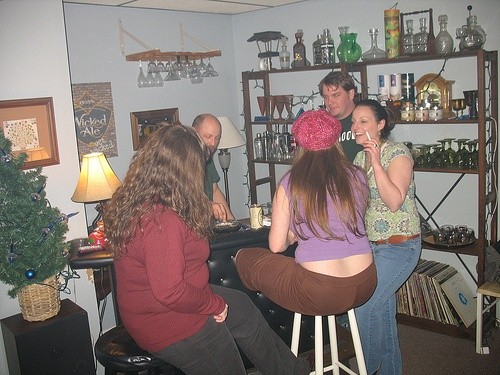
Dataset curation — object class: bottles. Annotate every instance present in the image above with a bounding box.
[376,72,415,104]
[402,15,483,55]
[279,36,290,70]
[254,124,298,161]
[385,101,444,120]
[291,25,386,67]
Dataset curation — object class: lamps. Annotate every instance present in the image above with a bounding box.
[216,117,246,209]
[71,152,123,229]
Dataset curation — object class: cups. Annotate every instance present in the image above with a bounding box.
[361,126,378,149]
[250,203,263,230]
[433,225,474,244]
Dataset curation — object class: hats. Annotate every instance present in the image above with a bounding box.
[292,109,341,152]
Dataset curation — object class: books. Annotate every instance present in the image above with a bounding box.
[395,259,478,328]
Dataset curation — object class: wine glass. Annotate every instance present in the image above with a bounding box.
[136,55,219,89]
[451,99,466,120]
[404,138,488,169]
[255,94,328,121]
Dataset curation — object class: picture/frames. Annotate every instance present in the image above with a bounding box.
[129,107,179,151]
[0,97,60,170]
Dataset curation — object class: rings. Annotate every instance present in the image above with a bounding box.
[374,144,377,147]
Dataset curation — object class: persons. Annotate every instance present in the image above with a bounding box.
[101,122,315,375]
[350,100,422,375]
[320,71,364,163]
[235,109,377,315]
[192,114,235,222]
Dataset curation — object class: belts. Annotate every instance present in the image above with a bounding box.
[370,234,419,244]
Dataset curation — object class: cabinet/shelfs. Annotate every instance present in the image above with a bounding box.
[241,50,498,348]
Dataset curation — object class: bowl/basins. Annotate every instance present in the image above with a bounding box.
[211,219,243,233]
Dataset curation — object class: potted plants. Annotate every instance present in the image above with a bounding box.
[0,126,72,322]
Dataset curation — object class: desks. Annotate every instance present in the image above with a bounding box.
[69,213,331,368]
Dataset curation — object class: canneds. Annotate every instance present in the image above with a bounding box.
[399,100,415,121]
[378,73,415,103]
[249,204,263,228]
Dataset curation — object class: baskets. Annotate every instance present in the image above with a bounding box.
[16,275,61,322]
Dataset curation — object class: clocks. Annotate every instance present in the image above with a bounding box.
[412,73,455,118]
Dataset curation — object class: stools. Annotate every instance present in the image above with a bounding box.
[94,325,169,375]
[290,308,367,375]
[476,281,500,355]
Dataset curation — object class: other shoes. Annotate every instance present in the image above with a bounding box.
[304,351,314,372]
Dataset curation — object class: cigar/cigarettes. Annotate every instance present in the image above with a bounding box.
[366,132,371,140]
